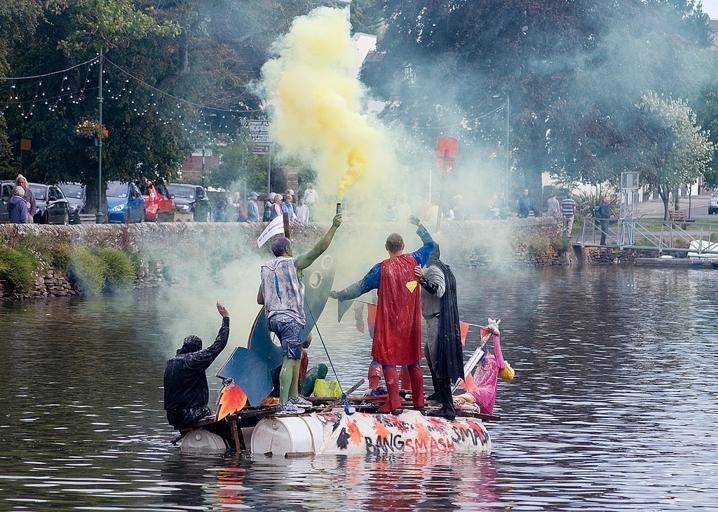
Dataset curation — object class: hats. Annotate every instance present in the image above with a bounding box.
[249,191,260,198]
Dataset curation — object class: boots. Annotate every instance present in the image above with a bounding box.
[366,364,458,419]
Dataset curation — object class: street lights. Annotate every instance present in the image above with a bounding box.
[491,89,512,214]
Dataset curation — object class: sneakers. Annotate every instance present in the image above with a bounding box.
[288,396,313,408]
[274,399,305,414]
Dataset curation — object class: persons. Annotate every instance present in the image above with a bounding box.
[546,191,559,216]
[11,173,35,216]
[263,192,311,222]
[561,193,576,238]
[256,213,342,414]
[273,333,328,397]
[414,241,467,420]
[487,190,511,219]
[9,186,29,223]
[519,188,531,217]
[164,300,248,451]
[304,184,320,208]
[597,197,611,244]
[223,191,258,223]
[331,215,434,413]
[440,192,463,219]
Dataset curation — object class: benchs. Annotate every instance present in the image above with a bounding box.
[668,209,696,230]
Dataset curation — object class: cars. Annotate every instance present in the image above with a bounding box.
[0,176,210,225]
[708,189,718,216]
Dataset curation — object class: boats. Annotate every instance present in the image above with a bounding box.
[172,395,498,459]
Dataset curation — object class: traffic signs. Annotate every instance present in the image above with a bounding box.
[248,133,273,143]
[247,119,273,134]
[251,143,271,155]
